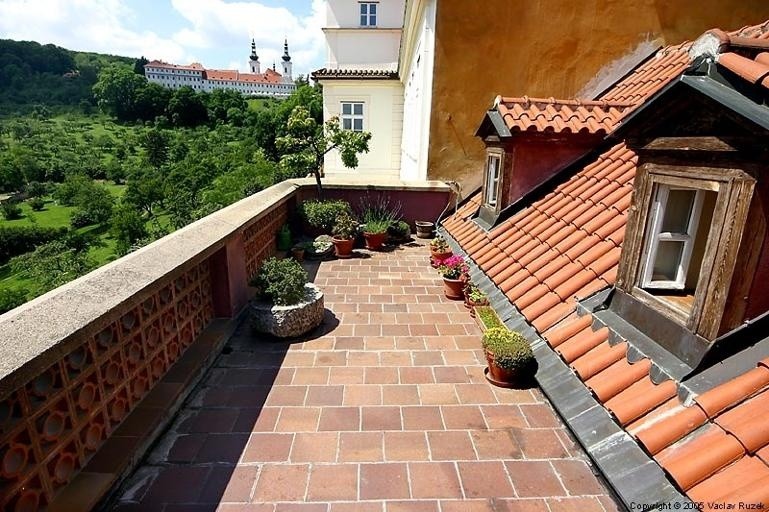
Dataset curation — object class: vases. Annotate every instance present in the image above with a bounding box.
[443,279,467,300]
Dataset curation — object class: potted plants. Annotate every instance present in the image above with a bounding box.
[246,256,325,339]
[462,283,510,333]
[430,235,453,267]
[482,327,538,388]
[290,196,411,262]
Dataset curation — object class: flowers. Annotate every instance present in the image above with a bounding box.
[433,256,471,279]
